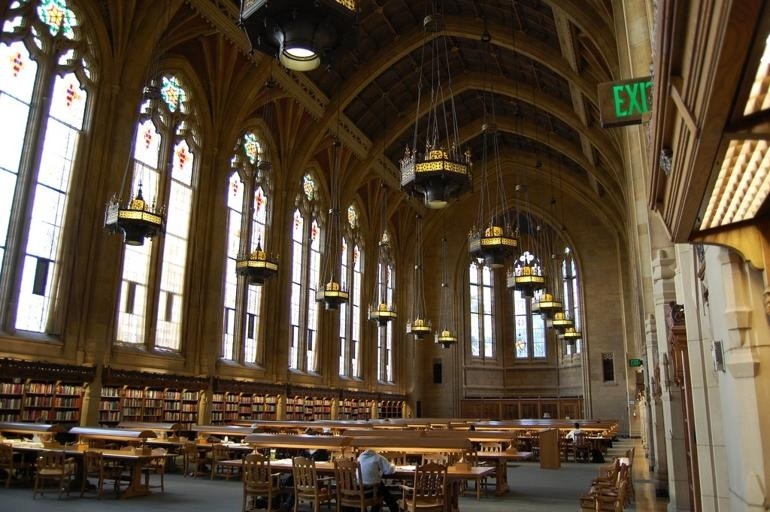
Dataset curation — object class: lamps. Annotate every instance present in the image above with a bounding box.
[513,105,583,345]
[235,0,364,73]
[235,58,280,287]
[316,91,458,350]
[105,1,168,245]
[395,4,519,271]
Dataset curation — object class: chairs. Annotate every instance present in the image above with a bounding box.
[577,445,637,512]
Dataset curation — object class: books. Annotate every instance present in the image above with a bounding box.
[1,382,407,427]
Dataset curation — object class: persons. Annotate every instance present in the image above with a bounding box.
[466,423,481,451]
[355,449,400,510]
[424,422,434,431]
[566,422,588,463]
[281,445,331,509]
